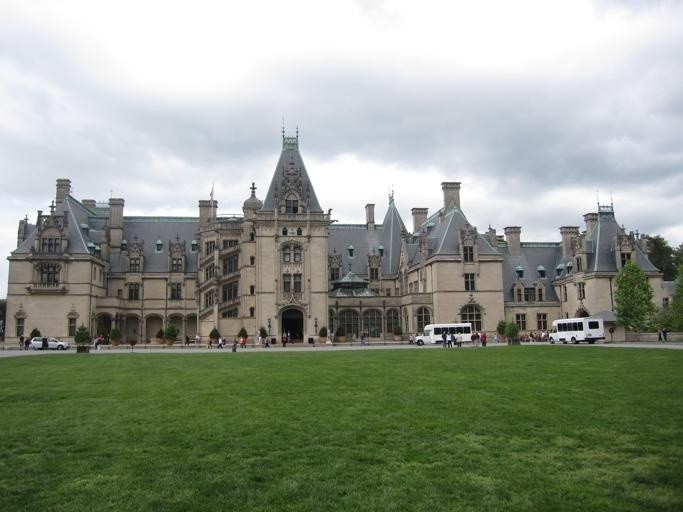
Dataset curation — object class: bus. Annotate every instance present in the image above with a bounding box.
[414,322,473,345]
[549,317,606,344]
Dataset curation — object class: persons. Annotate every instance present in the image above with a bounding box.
[283,336,287,347]
[471,331,487,347]
[658,328,666,341]
[442,331,462,348]
[264,336,269,348]
[185,334,226,349]
[20,335,30,350]
[240,335,246,348]
[42,336,48,349]
[94,334,104,350]
[520,329,556,342]
[409,334,414,344]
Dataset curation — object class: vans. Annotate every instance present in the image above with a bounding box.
[30,337,70,351]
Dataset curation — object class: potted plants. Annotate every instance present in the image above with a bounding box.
[237,327,248,345]
[111,328,122,345]
[504,323,520,346]
[74,321,91,353]
[259,327,268,344]
[163,326,179,346]
[336,328,345,342]
[496,320,506,342]
[391,326,402,342]
[209,327,220,345]
[319,326,327,344]
